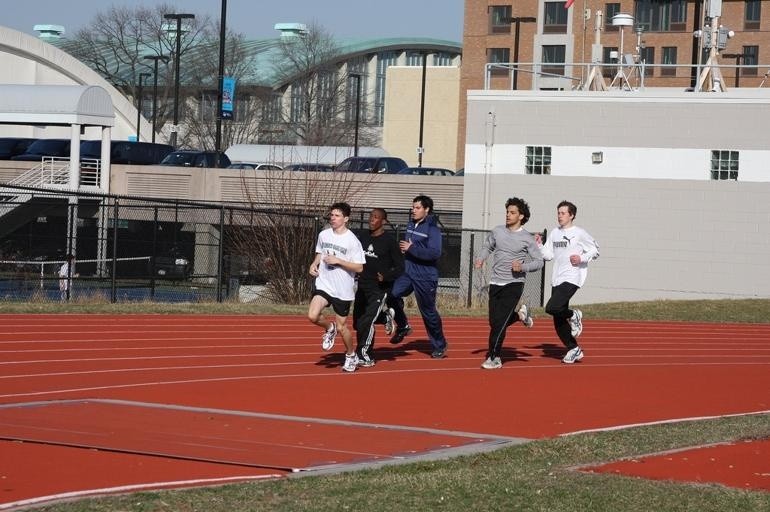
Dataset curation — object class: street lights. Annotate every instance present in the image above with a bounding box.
[634,26,643,90]
[136,73,148,140]
[163,12,196,149]
[501,16,537,90]
[144,55,171,142]
[410,47,443,170]
[611,12,635,88]
[346,74,360,158]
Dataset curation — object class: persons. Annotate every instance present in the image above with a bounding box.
[474,196,545,370]
[306,202,367,374]
[58,253,80,301]
[533,200,601,365]
[352,206,406,367]
[384,194,450,360]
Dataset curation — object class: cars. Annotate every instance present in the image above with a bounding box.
[1,228,297,283]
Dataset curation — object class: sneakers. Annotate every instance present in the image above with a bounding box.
[322,321,338,352]
[480,356,504,369]
[518,304,533,329]
[358,357,376,368]
[565,308,585,339]
[341,352,360,372]
[431,342,449,358]
[384,307,396,336]
[390,324,413,344]
[561,345,584,364]
